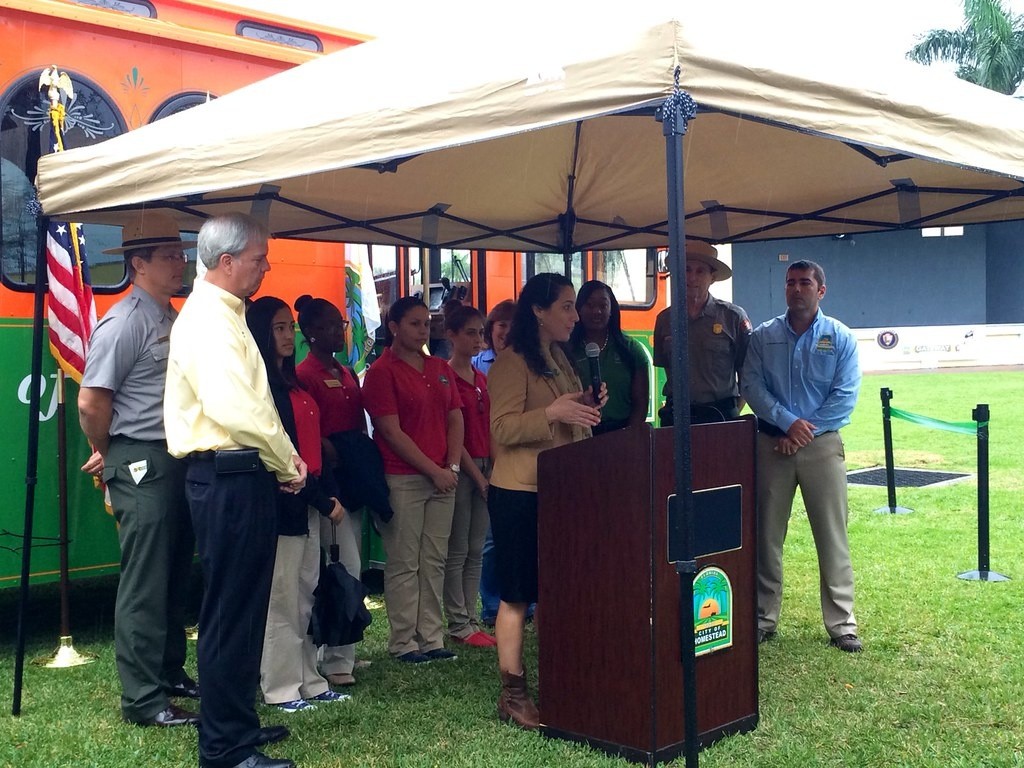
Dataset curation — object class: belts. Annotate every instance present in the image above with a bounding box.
[690,397,737,415]
[191,451,214,460]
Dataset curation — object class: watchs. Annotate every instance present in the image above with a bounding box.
[445,463,460,473]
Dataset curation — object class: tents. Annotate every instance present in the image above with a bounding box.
[12,0,1024,768]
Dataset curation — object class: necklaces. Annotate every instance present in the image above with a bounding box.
[582,328,609,352]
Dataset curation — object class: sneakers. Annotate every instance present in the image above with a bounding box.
[260,695,318,713]
[302,691,351,703]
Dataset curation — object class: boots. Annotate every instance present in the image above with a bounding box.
[497,664,539,732]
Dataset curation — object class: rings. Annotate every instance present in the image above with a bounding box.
[98,471,102,474]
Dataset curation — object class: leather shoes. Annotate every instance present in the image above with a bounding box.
[165,678,200,697]
[199,753,293,768]
[138,703,199,727]
[255,726,288,747]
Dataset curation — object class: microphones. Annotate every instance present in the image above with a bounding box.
[585,342,602,405]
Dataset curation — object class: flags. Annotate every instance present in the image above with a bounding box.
[47,110,98,387]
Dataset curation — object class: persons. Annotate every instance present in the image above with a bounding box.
[163,211,308,768]
[739,261,862,653]
[245,294,536,714]
[78,212,202,728]
[487,272,608,731]
[653,239,753,427]
[559,280,650,436]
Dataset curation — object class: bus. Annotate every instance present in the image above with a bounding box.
[0,0,670,592]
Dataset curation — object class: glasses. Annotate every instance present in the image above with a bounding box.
[168,254,188,262]
[312,320,349,335]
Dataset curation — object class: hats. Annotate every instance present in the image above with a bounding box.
[665,241,731,281]
[102,214,198,255]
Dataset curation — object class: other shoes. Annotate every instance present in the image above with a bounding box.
[465,632,497,646]
[423,649,458,661]
[397,652,431,665]
[830,635,862,652]
[758,629,774,643]
